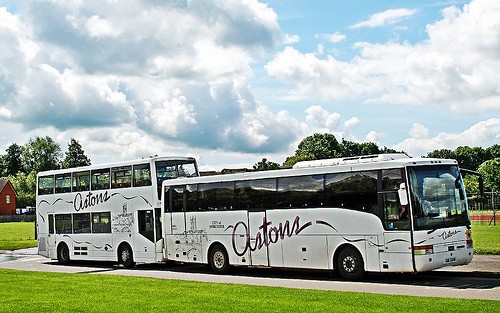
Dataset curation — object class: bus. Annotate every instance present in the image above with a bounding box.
[35,154,202,269]
[159,152,485,282]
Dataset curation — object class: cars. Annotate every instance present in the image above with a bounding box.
[16,206,35,215]
